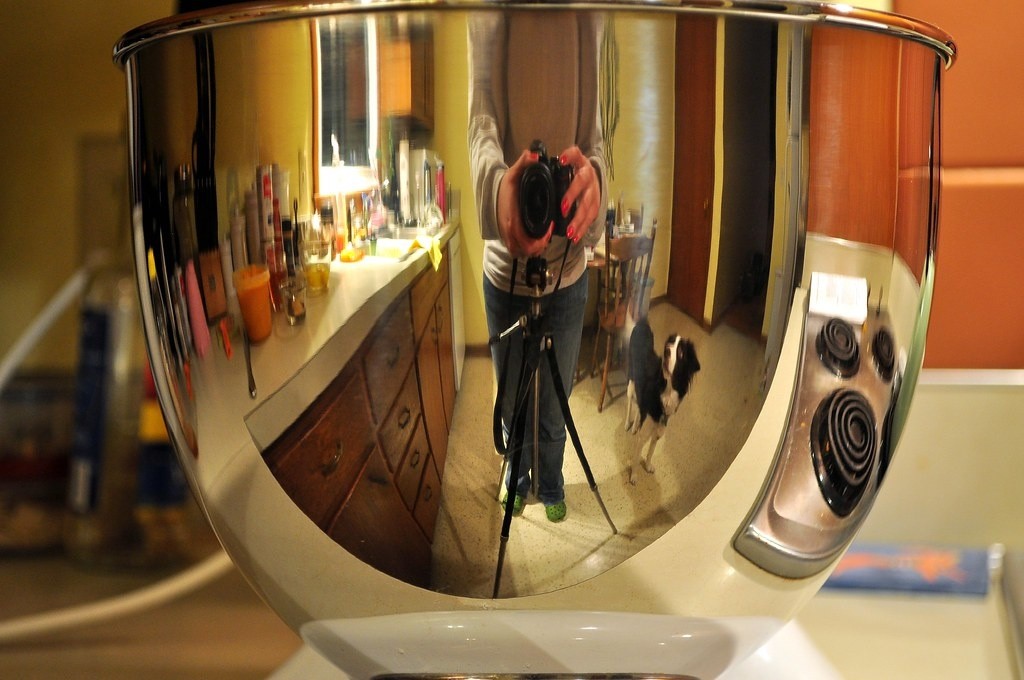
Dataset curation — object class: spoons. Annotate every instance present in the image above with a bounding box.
[240,327,256,398]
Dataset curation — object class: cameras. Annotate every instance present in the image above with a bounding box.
[520,142,579,238]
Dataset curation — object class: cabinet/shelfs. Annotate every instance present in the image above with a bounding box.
[260,236,463,583]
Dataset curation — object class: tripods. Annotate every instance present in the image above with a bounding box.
[484,256,618,600]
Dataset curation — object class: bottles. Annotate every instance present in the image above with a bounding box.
[66,180,147,562]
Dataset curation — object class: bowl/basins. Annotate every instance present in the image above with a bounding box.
[115,1,956,678]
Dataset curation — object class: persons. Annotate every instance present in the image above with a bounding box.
[464,7,608,521]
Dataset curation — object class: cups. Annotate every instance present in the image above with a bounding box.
[298,236,331,300]
[232,262,273,341]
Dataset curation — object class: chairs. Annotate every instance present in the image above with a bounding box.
[588,215,661,412]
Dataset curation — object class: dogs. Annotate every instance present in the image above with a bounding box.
[622,300,700,485]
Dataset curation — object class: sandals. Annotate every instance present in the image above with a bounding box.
[545,499,566,521]
[502,491,523,514]
